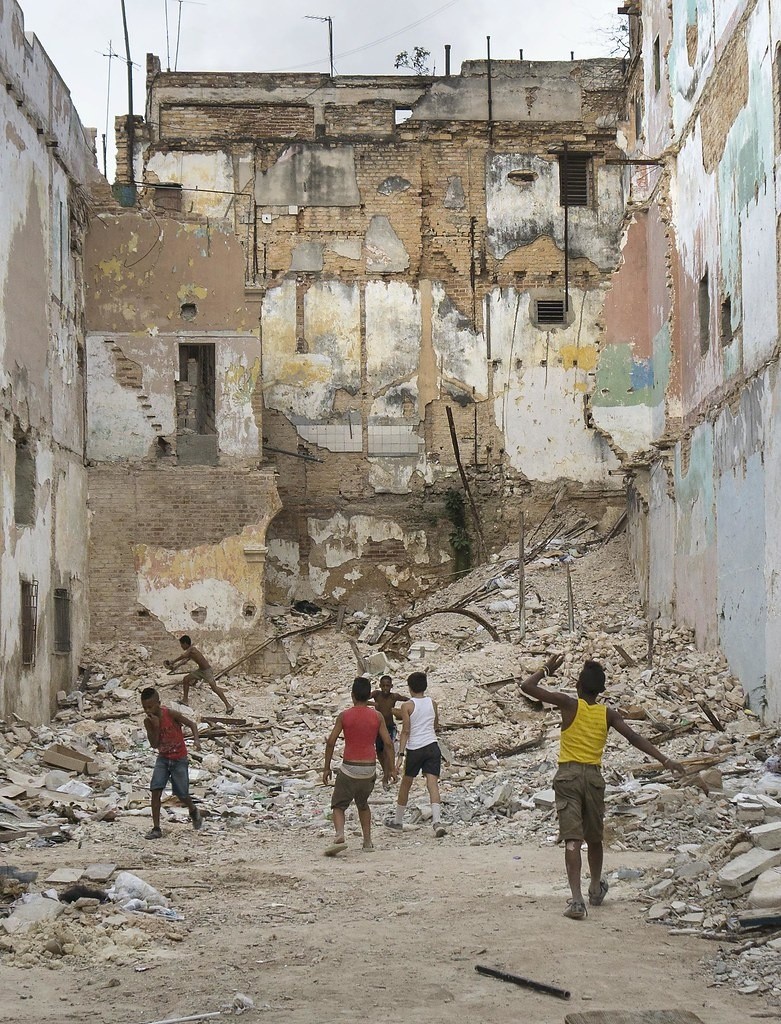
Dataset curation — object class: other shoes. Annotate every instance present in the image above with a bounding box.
[383,819,403,833]
[563,898,588,918]
[588,878,608,906]
[433,822,447,837]
[189,805,201,830]
[145,828,162,839]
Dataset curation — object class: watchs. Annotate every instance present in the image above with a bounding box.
[398,752,404,756]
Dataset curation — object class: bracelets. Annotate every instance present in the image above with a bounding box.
[539,665,550,678]
[663,758,670,765]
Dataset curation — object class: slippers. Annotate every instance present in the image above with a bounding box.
[324,841,348,856]
[362,843,375,851]
[177,701,188,706]
[226,708,234,715]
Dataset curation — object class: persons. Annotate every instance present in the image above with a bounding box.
[170,635,234,715]
[520,653,687,919]
[322,672,448,855]
[141,687,203,840]
